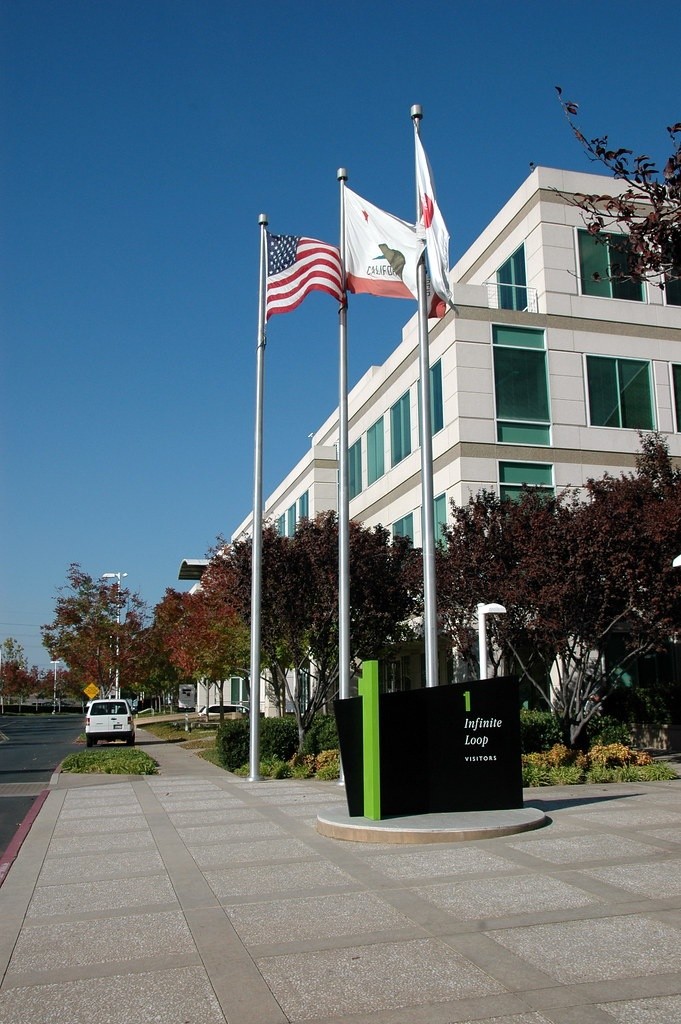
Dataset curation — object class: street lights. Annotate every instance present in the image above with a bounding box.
[49,661,60,716]
[103,572,129,713]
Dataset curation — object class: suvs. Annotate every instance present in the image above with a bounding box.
[84,699,139,747]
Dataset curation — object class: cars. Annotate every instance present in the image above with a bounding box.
[197,705,249,721]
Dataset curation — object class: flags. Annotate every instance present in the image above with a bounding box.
[415,125,452,304]
[266,231,346,322]
[344,184,447,319]
